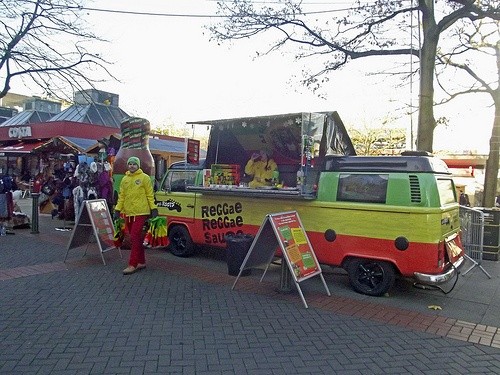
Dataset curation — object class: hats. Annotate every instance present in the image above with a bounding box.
[127,157,140,169]
[52,161,113,176]
[260,146,274,157]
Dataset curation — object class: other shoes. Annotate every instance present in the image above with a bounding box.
[122,265,139,275]
[137,263,146,269]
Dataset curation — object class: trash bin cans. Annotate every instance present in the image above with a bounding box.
[225,234,254,276]
[469,207,500,261]
[0,192,12,222]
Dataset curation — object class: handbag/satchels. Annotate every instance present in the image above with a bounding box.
[143,216,170,250]
[113,218,130,248]
[40,181,56,197]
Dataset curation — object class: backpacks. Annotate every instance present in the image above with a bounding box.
[465,194,469,205]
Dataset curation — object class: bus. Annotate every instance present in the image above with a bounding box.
[155,154,466,297]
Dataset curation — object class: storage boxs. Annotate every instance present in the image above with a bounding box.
[211,163,241,184]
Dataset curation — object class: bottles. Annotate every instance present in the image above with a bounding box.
[111,116,156,250]
[217,176,234,186]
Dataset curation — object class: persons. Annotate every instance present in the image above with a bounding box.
[115,156,158,275]
[244,147,279,188]
[459,190,471,207]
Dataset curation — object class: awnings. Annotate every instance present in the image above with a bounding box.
[0,137,53,154]
[59,136,187,155]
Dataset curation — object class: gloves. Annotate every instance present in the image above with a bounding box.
[112,210,120,221]
[151,209,159,218]
